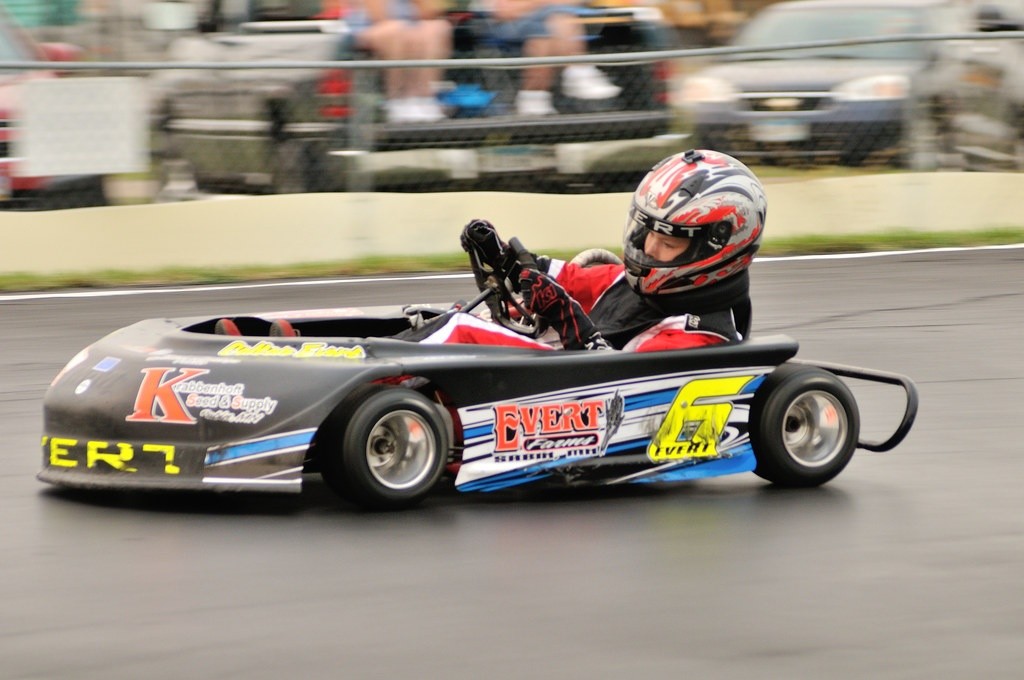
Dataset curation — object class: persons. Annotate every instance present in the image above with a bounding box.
[461,149,766,351]
[468,1,622,115]
[353,0,452,122]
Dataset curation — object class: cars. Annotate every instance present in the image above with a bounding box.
[689,0,978,168]
[907,41,1024,172]
[0,13,107,210]
[166,22,388,192]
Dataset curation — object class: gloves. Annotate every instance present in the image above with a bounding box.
[460,219,552,294]
[518,268,602,350]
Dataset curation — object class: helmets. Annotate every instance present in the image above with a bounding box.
[624,149,766,299]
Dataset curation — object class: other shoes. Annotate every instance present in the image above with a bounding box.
[384,98,446,122]
[560,63,622,99]
[514,89,559,115]
